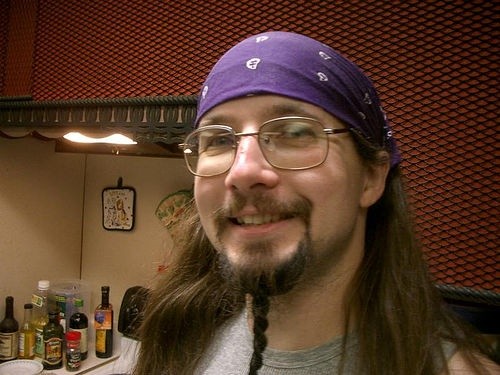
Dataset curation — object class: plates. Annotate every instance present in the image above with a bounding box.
[0,360,44,375]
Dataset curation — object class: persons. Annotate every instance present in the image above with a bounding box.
[110,30,500,375]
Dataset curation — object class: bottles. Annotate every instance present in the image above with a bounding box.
[0,296,18,362]
[93,285,113,359]
[65,330,82,371]
[69,299,88,360]
[55,295,59,317]
[42,310,64,370]
[31,280,51,320]
[57,297,67,335]
[17,303,36,360]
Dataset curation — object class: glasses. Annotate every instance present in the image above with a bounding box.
[177,116,351,177]
